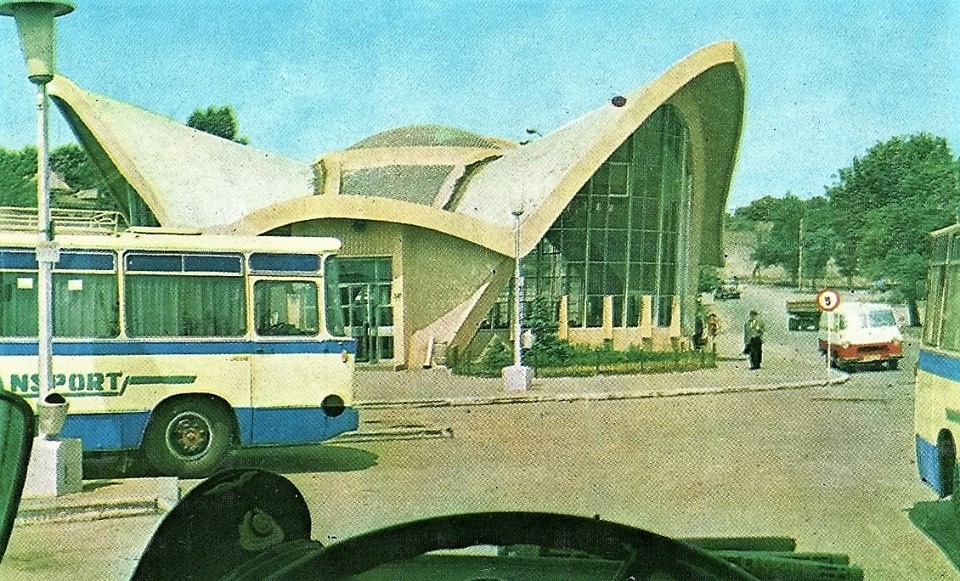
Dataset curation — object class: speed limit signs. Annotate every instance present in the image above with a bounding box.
[816,287,840,312]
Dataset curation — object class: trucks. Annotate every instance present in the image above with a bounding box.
[786,298,821,332]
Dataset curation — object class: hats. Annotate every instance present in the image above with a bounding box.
[750,310,759,316]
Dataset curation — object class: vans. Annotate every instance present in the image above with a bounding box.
[817,302,903,372]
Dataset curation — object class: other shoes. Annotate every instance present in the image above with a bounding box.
[750,366,758,369]
[758,363,761,369]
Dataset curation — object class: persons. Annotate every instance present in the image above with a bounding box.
[744,310,767,370]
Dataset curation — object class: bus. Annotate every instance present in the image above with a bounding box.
[0,208,359,480]
[913,224,960,520]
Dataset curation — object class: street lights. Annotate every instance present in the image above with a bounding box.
[0,0,84,497]
[501,188,532,393]
